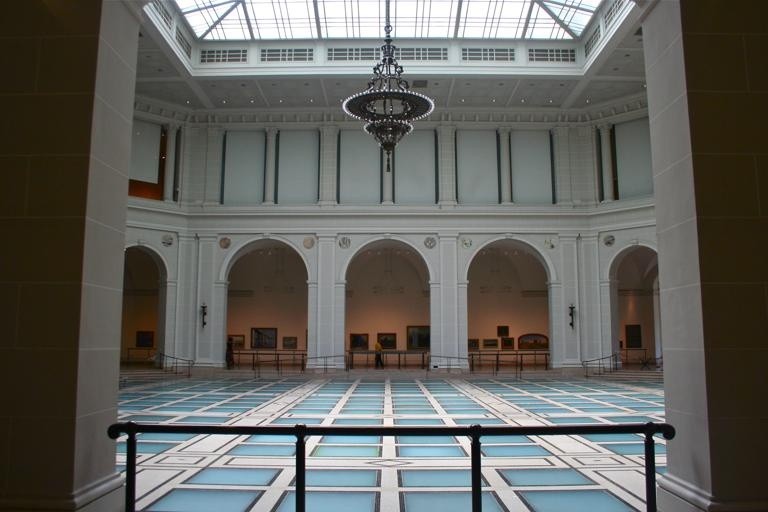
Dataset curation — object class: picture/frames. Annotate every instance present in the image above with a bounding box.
[625,324,642,348]
[350,326,429,351]
[468,326,548,351]
[227,328,308,350]
[136,331,154,347]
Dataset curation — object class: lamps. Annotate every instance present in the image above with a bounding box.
[341,0,435,171]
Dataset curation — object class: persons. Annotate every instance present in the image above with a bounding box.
[374,338,385,370]
